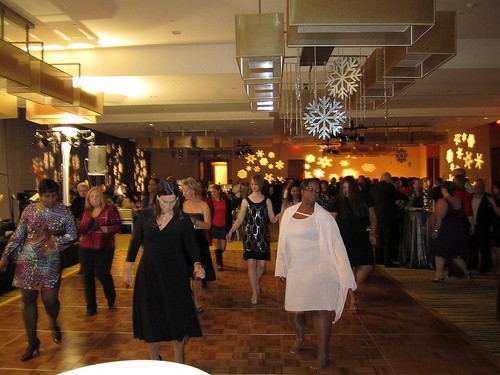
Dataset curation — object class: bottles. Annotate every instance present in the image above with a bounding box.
[432,200,435,207]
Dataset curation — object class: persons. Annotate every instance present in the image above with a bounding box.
[226,173,284,304]
[125,180,206,363]
[78,187,122,315]
[101,169,500,285]
[70,182,89,221]
[275,179,358,369]
[329,175,377,313]
[0,179,78,361]
[179,177,212,313]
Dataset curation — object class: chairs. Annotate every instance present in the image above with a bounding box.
[117,208,133,235]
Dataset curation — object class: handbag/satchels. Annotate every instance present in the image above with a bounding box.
[80,231,103,250]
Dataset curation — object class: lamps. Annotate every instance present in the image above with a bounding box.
[31,128,95,148]
[234,144,254,156]
[317,120,368,155]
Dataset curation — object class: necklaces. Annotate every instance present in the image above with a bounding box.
[162,212,171,220]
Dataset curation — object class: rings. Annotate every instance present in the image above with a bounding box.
[202,276,204,279]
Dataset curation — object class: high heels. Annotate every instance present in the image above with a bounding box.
[52,329,60,344]
[21,339,40,362]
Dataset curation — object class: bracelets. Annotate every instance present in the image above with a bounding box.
[434,229,439,232]
[194,262,201,267]
[193,218,197,226]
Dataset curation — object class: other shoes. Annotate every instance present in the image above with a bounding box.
[432,274,445,281]
[218,264,223,271]
[309,357,327,370]
[385,262,400,268]
[109,298,115,307]
[257,286,260,293]
[251,297,258,305]
[196,307,202,313]
[350,303,359,315]
[86,308,96,316]
[289,336,304,354]
